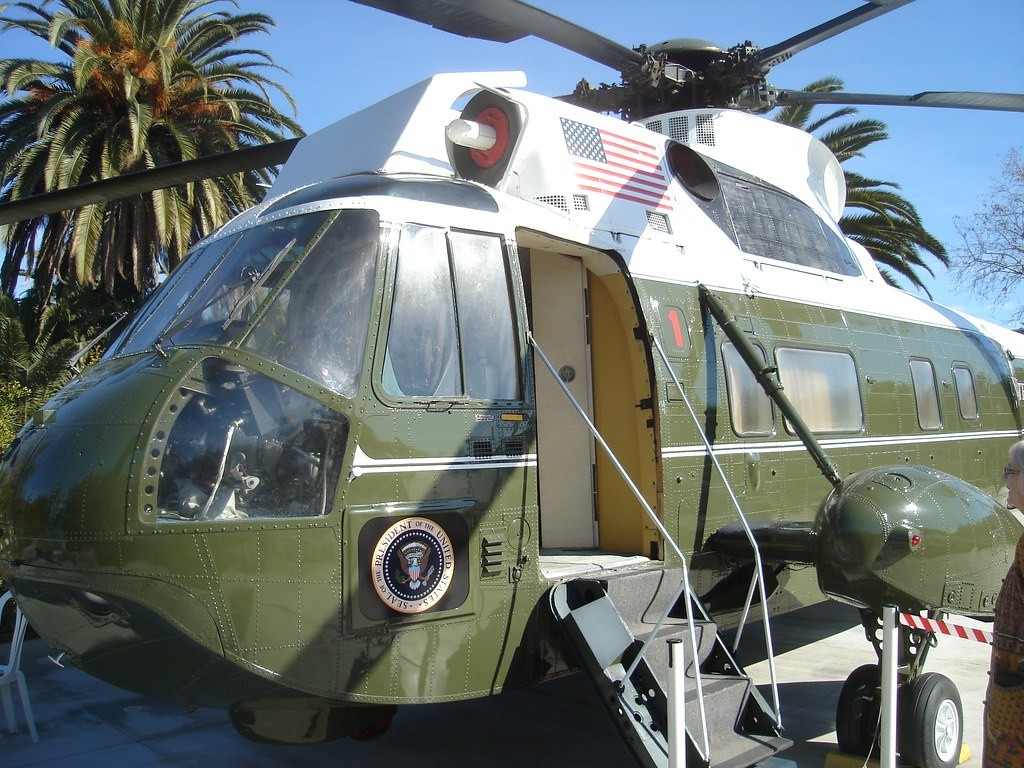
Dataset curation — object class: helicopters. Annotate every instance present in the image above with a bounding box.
[0,1,1022,768]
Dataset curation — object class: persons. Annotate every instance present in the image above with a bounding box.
[983,443,1024,768]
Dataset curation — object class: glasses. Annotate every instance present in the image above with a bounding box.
[1004,467,1021,476]
